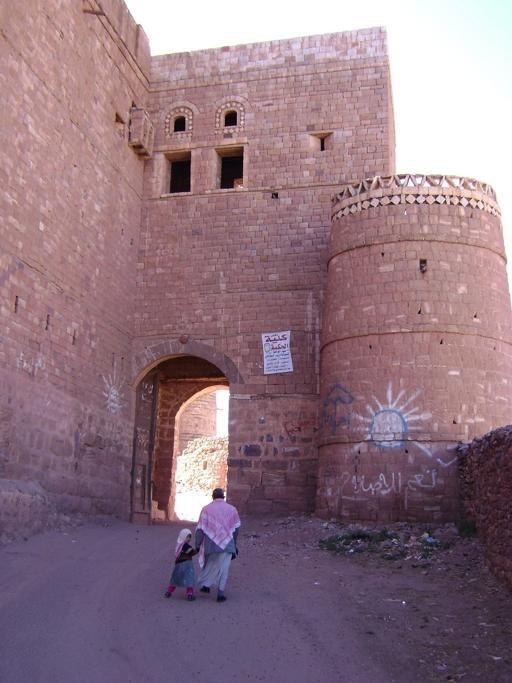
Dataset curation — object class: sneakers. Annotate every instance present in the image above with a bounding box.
[164,591,172,598]
[217,595,227,603]
[185,586,210,601]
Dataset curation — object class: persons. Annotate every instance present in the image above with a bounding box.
[162,528,199,601]
[194,487,242,602]
